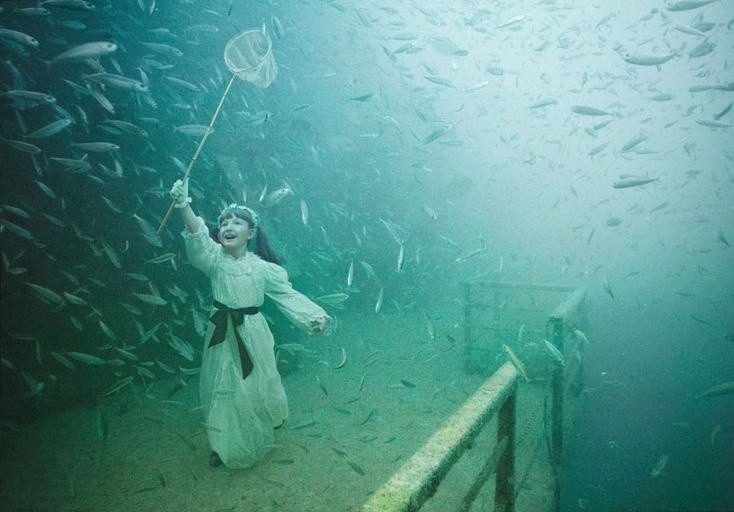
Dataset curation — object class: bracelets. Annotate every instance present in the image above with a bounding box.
[175,198,191,209]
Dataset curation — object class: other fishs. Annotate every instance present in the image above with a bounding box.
[1,0,734,512]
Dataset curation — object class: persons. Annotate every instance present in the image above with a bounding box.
[171,178,328,470]
